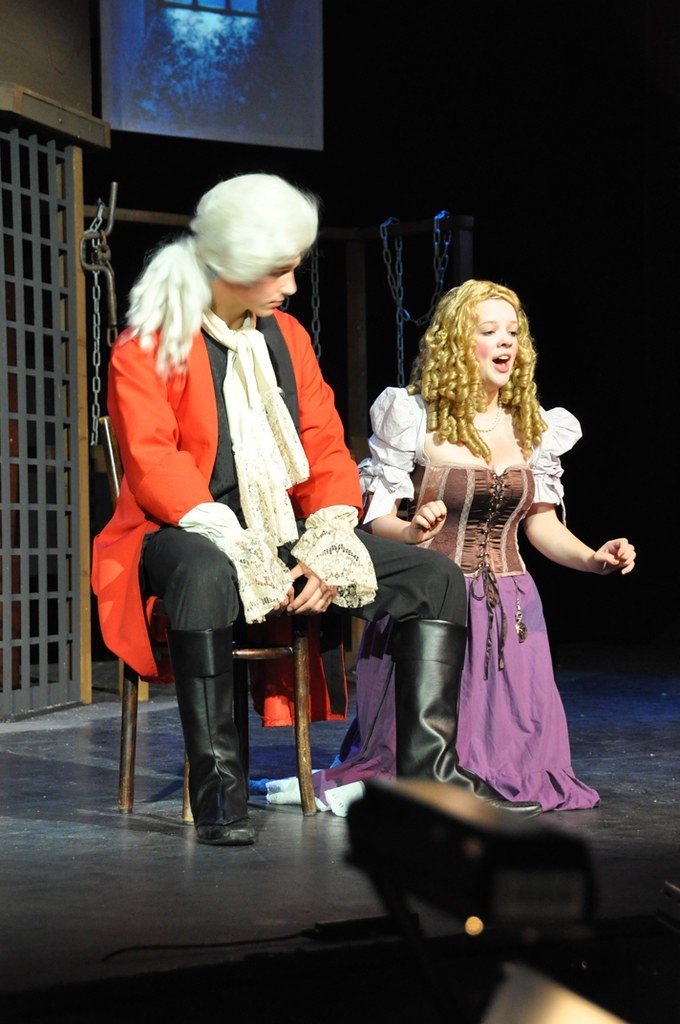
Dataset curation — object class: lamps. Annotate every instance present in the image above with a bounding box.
[347,778,596,934]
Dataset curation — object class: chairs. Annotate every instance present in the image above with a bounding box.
[101,415,317,821]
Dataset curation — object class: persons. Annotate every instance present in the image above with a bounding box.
[265,279,637,814]
[93,173,540,848]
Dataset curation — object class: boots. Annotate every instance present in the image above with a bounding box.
[385,616,544,820]
[165,622,256,847]
